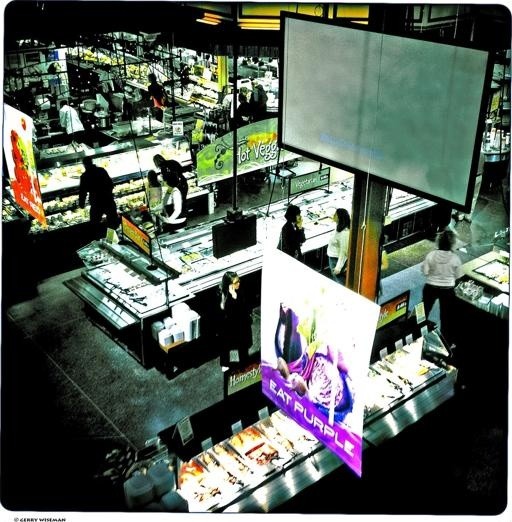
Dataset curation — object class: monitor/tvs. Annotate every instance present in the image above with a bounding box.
[212,213,257,260]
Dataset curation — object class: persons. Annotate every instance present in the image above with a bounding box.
[148,173,186,233]
[276,205,307,262]
[326,208,351,286]
[237,92,251,124]
[147,73,170,124]
[217,271,254,373]
[451,152,487,223]
[153,153,195,219]
[250,79,268,123]
[58,99,85,142]
[421,230,465,342]
[78,156,117,240]
[144,170,164,234]
